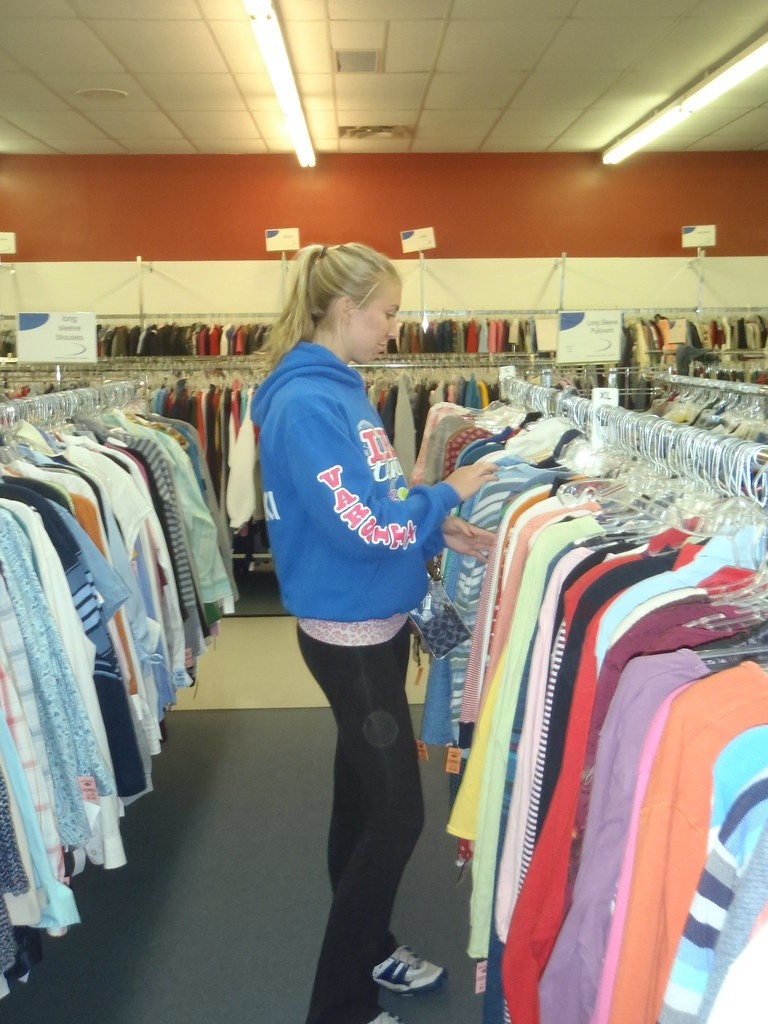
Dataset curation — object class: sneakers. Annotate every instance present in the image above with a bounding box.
[369,1011,408,1024]
[371,941,447,998]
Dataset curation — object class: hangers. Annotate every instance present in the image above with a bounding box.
[362,352,768,673]
[0,355,256,463]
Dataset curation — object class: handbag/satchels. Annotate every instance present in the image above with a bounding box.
[402,574,475,662]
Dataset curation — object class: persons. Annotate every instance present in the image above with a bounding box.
[250,243,501,1024]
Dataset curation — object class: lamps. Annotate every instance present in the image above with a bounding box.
[244,0,316,168]
[602,32,768,165]
